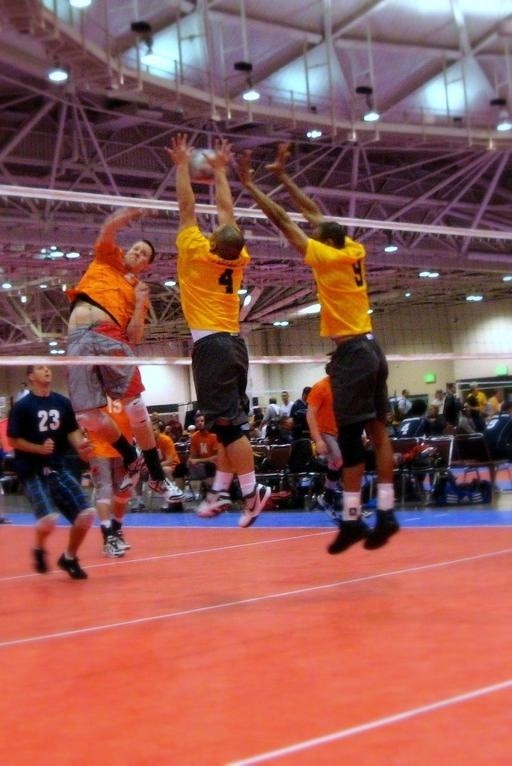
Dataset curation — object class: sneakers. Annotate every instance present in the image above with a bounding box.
[102,526,131,558]
[327,515,373,554]
[130,474,204,512]
[238,482,272,528]
[32,548,47,573]
[57,553,87,579]
[196,491,231,519]
[316,491,338,519]
[365,508,400,550]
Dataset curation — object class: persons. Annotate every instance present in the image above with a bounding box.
[236,141,399,552]
[15,382,30,403]
[63,208,185,504]
[85,393,133,557]
[6,365,97,580]
[164,133,272,529]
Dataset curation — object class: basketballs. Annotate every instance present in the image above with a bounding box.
[189,149,223,181]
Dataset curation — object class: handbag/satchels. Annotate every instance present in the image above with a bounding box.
[434,471,493,506]
[264,489,299,511]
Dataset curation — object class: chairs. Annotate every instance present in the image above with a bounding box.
[1,416,24,496]
[148,408,329,515]
[363,413,510,512]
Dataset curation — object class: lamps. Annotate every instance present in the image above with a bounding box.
[232,58,262,102]
[128,19,164,67]
[353,85,381,122]
[487,94,512,132]
[303,104,324,140]
